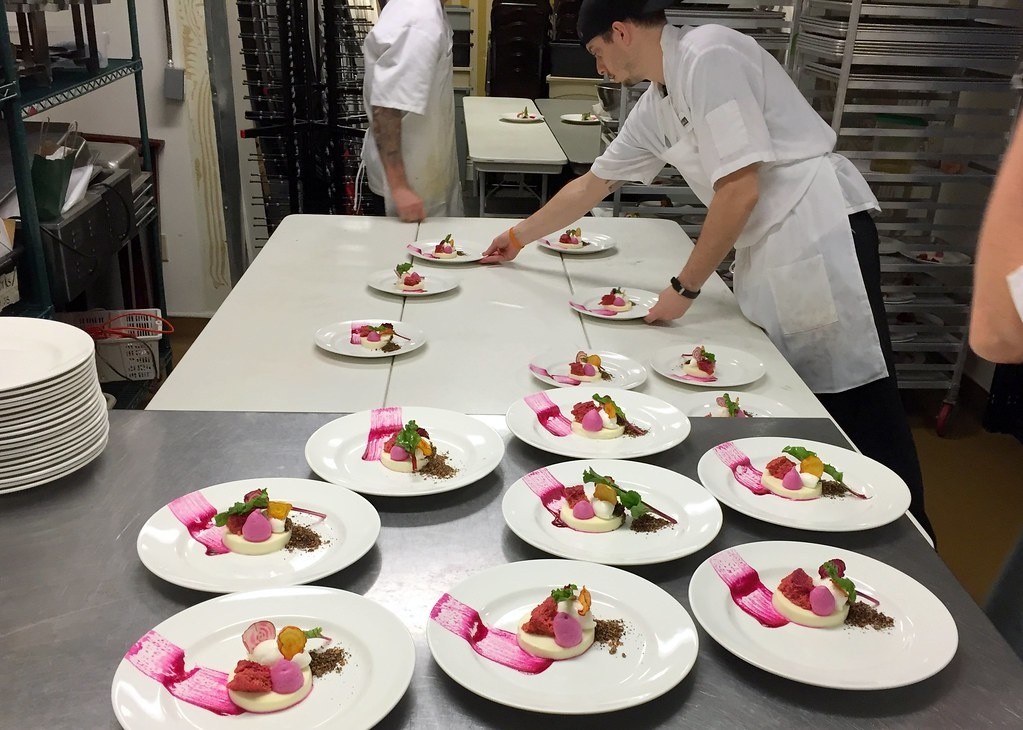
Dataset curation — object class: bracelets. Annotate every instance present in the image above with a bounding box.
[509,227,522,249]
[670,277,700,299]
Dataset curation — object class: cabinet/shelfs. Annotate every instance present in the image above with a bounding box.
[0,0,174,411]
[598,1,1023,434]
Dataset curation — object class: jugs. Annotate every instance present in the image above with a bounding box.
[870,112,928,175]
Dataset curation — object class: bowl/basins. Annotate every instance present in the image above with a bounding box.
[594,82,621,121]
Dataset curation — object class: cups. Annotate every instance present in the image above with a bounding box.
[939,126,976,175]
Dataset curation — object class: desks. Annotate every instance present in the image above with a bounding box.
[462,96,568,218]
[534,98,602,163]
[144,215,935,550]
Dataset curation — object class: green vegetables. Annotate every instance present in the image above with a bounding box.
[393,419,424,475]
[681,351,716,364]
[366,325,410,340]
[301,627,332,640]
[783,446,865,497]
[723,392,741,419]
[823,562,880,604]
[582,467,677,523]
[549,584,579,604]
[591,394,645,436]
[444,234,452,242]
[611,287,622,293]
[214,488,327,527]
[396,262,412,274]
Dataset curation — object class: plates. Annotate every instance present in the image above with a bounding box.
[136,477,382,595]
[304,405,504,497]
[560,113,599,123]
[505,386,692,460]
[366,267,459,297]
[501,459,724,566]
[0,316,110,495]
[875,233,973,384]
[313,318,429,358]
[501,112,543,122]
[115,588,417,730]
[687,540,962,690]
[537,231,619,253]
[425,559,701,716]
[405,238,490,265]
[567,286,659,320]
[648,342,767,387]
[528,349,648,390]
[697,436,912,534]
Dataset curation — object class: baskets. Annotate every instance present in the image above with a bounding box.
[47,307,162,383]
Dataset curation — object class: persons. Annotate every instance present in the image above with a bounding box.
[966,115,1022,663]
[480,0,934,548]
[360,0,464,222]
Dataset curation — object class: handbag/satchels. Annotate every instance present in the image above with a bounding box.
[31,116,78,222]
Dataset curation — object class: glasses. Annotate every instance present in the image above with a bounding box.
[576,0,684,47]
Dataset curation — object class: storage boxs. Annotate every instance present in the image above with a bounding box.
[54,308,162,383]
